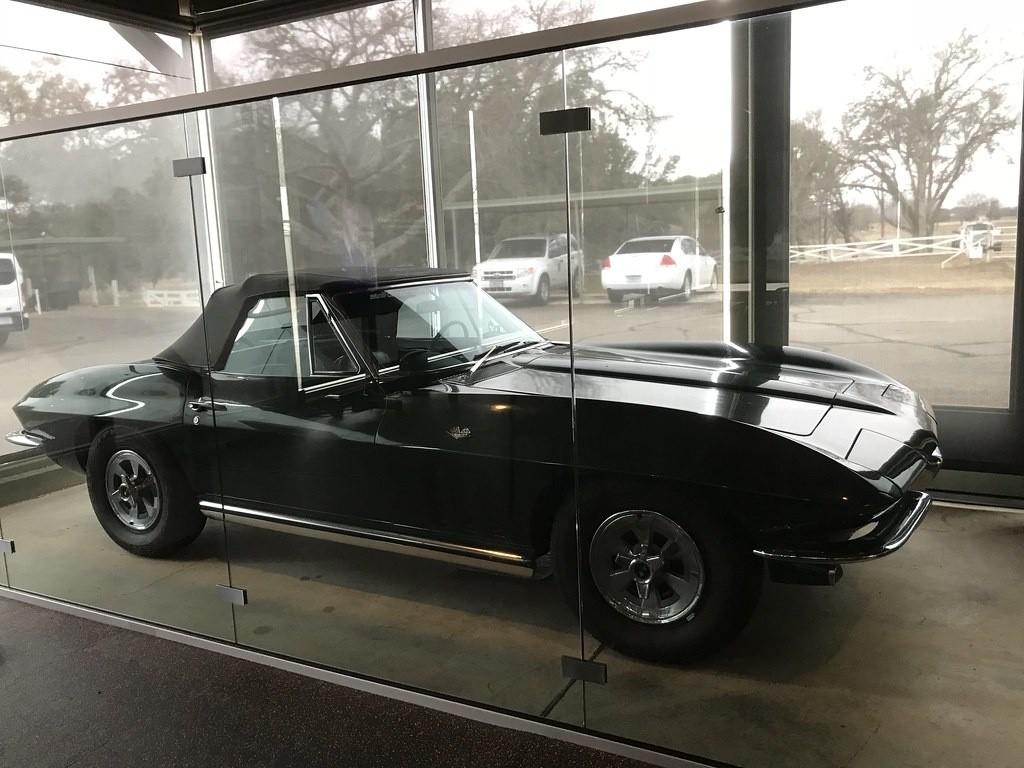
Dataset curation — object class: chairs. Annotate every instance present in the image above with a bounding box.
[330,352,391,370]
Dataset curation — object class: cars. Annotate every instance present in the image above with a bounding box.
[598,232,719,303]
[470,232,587,304]
[0,250,31,348]
[11,265,948,667]
